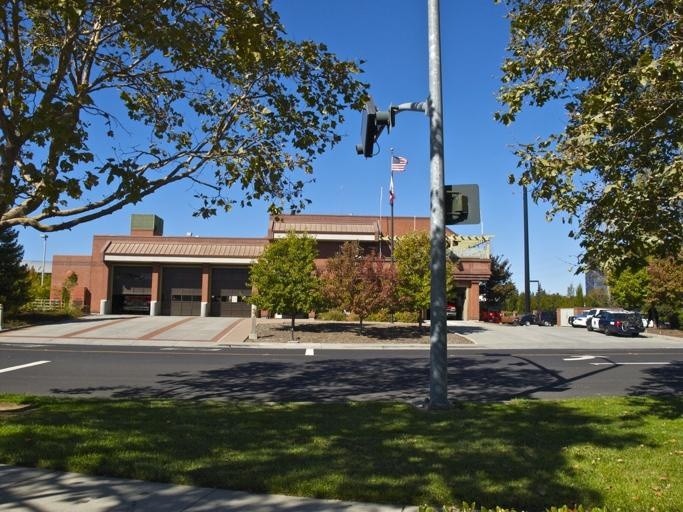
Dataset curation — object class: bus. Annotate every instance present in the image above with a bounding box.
[498,309,539,324]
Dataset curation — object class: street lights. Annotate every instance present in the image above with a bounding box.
[40,235,48,286]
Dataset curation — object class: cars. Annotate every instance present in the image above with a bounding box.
[568,307,653,336]
[510,314,543,326]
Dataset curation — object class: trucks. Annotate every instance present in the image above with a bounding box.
[533,312,557,327]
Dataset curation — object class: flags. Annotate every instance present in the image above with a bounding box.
[391,156,408,171]
[389,177,396,206]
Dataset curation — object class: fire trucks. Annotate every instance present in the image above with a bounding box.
[446,300,456,318]
[123,297,150,312]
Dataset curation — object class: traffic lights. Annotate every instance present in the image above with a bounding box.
[374,101,395,136]
[355,144,367,161]
